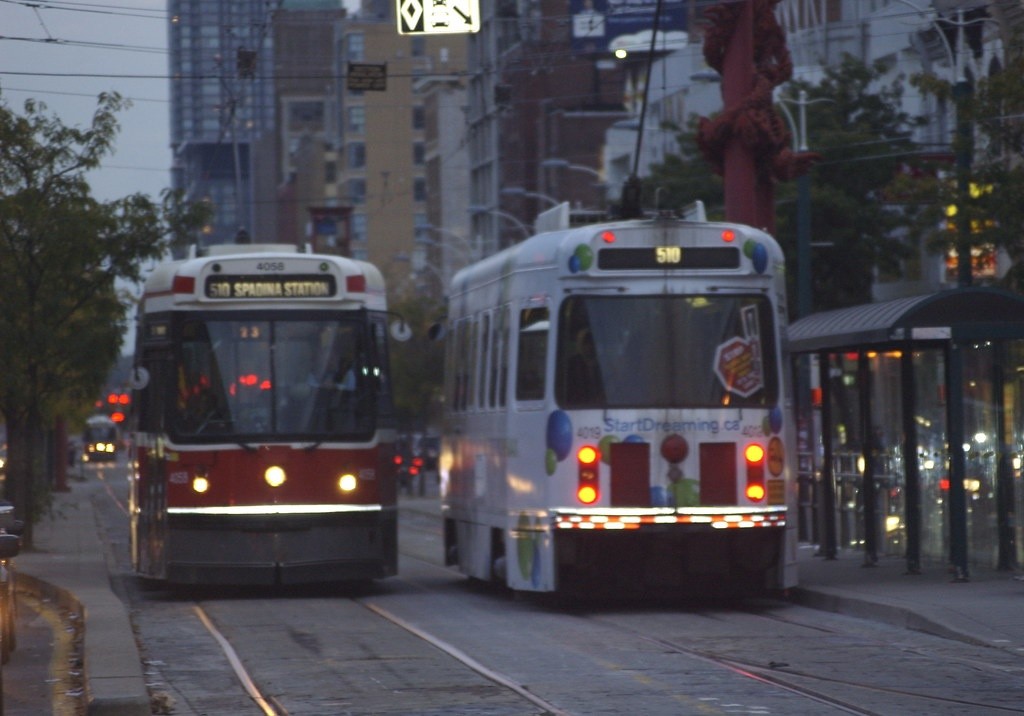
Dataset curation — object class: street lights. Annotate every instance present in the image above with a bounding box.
[687,70,841,561]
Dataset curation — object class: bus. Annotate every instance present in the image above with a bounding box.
[129,242,412,594]
[82,415,121,462]
[437,200,800,598]
